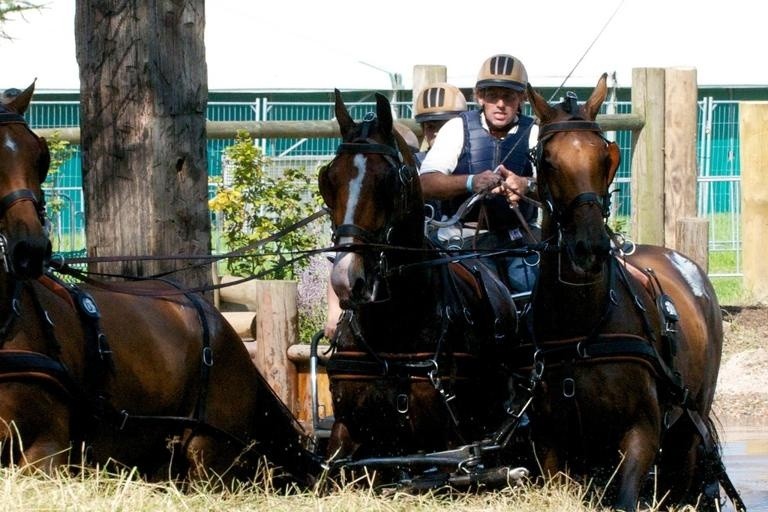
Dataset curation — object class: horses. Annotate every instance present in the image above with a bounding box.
[0,78,317,496]
[519,72,724,512]
[317,87,520,493]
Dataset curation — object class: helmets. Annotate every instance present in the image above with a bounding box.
[473,53,530,95]
[410,81,470,123]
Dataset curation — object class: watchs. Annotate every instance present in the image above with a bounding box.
[526,176,536,195]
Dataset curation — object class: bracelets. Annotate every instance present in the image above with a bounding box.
[466,174,475,193]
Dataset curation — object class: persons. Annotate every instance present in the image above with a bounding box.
[410,83,467,171]
[417,53,543,296]
[324,271,343,343]
